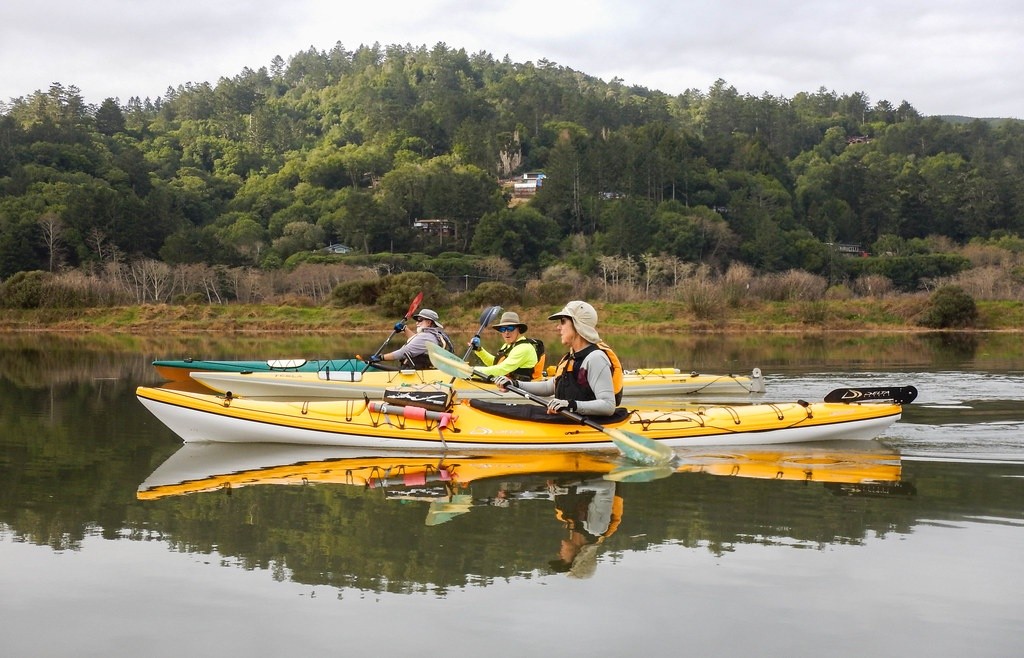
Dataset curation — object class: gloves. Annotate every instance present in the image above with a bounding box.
[368,354,384,364]
[394,323,407,333]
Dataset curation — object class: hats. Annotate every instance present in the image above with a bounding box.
[492,311,528,334]
[547,300,600,344]
[412,308,444,329]
[548,545,599,580]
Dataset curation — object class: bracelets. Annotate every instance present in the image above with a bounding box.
[511,379,519,388]
[568,399,577,413]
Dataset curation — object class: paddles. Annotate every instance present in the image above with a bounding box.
[425,467,675,527]
[360,291,424,375]
[449,305,505,385]
[427,340,680,466]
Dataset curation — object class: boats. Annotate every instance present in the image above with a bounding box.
[147,358,407,384]
[187,370,769,400]
[135,442,912,511]
[136,385,924,444]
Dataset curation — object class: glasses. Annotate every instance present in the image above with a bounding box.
[415,317,428,322]
[498,325,519,333]
[561,316,571,325]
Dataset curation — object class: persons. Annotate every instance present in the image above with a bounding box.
[467,312,538,382]
[546,478,616,572]
[368,309,447,370]
[494,300,616,415]
